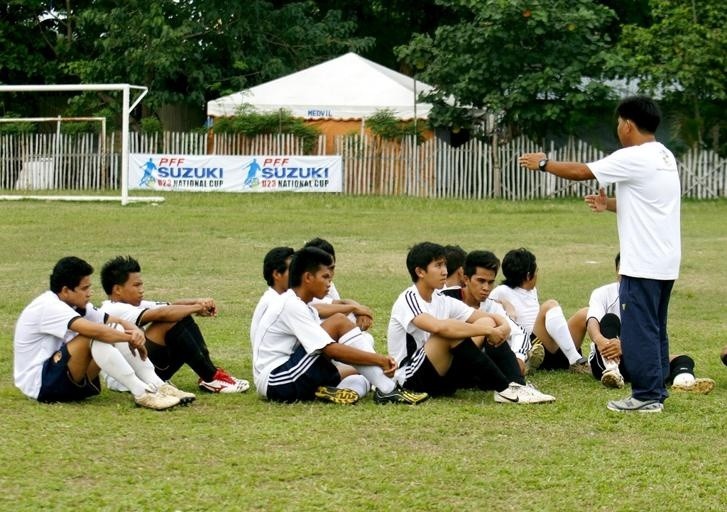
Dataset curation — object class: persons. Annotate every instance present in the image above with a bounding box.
[517,95,681,413]
[439,250,531,391]
[438,243,546,377]
[13,257,196,411]
[100,256,249,394]
[586,252,716,395]
[387,242,556,404]
[252,247,430,405]
[243,158,261,186]
[139,157,157,186]
[489,247,594,376]
[250,247,374,350]
[305,236,340,301]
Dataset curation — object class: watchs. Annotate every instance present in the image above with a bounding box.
[538,159,549,172]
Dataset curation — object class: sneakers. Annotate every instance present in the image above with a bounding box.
[198,367,250,394]
[525,344,545,376]
[315,384,358,404]
[373,380,429,405]
[607,396,662,413]
[601,367,624,388]
[671,378,715,395]
[493,380,556,404]
[569,358,592,373]
[134,384,195,411]
